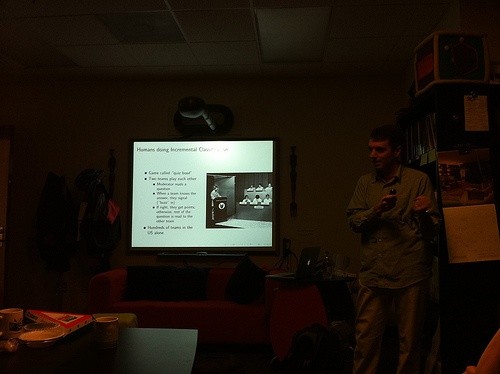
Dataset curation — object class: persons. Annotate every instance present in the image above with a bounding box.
[346,126,442,374]
[211,186,222,220]
[243,184,272,202]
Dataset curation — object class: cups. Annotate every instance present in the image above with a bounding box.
[0,308,24,339]
[94,316,119,349]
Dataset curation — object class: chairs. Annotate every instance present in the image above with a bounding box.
[119,329,199,374]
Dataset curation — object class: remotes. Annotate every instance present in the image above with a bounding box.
[390,188,397,195]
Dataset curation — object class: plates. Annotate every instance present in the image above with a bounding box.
[24,322,60,331]
[18,330,65,341]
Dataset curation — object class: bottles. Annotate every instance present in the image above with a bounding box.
[323,256,332,280]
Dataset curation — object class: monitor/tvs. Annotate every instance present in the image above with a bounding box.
[127,138,282,260]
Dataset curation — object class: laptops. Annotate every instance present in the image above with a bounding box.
[266,246,320,278]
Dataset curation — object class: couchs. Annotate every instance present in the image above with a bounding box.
[89,266,328,361]
